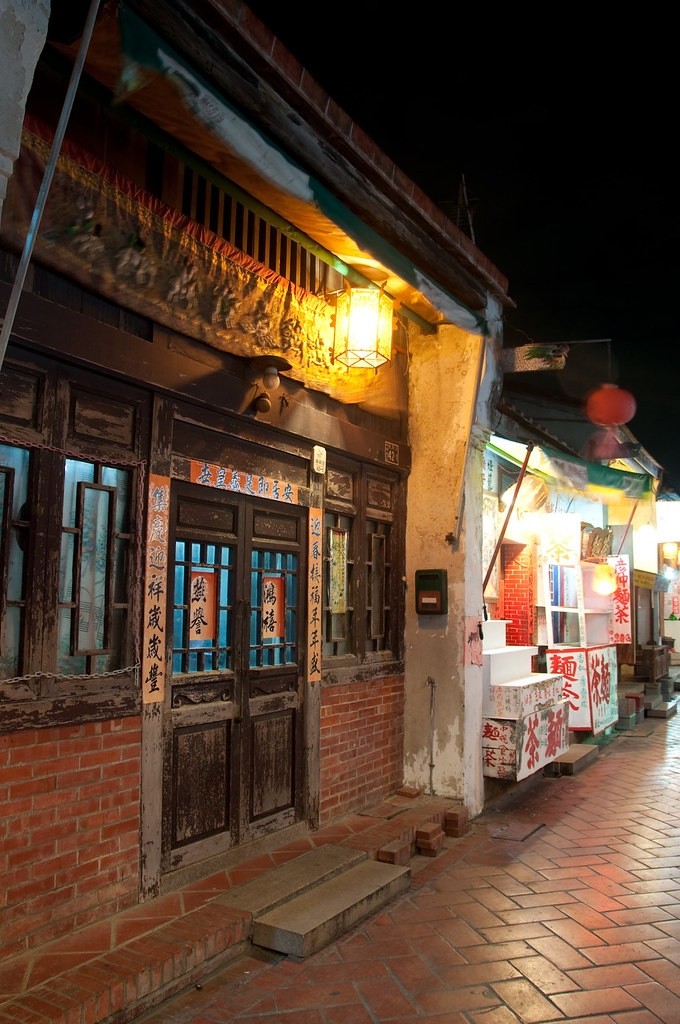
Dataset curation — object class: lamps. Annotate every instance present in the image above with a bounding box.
[252,355,293,389]
[328,279,394,376]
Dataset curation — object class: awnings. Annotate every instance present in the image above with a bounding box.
[484,429,653,594]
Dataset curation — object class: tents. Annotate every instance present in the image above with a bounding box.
[593,564,617,595]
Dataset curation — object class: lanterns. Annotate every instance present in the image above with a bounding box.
[662,543,677,558]
[332,278,394,375]
[586,384,636,445]
